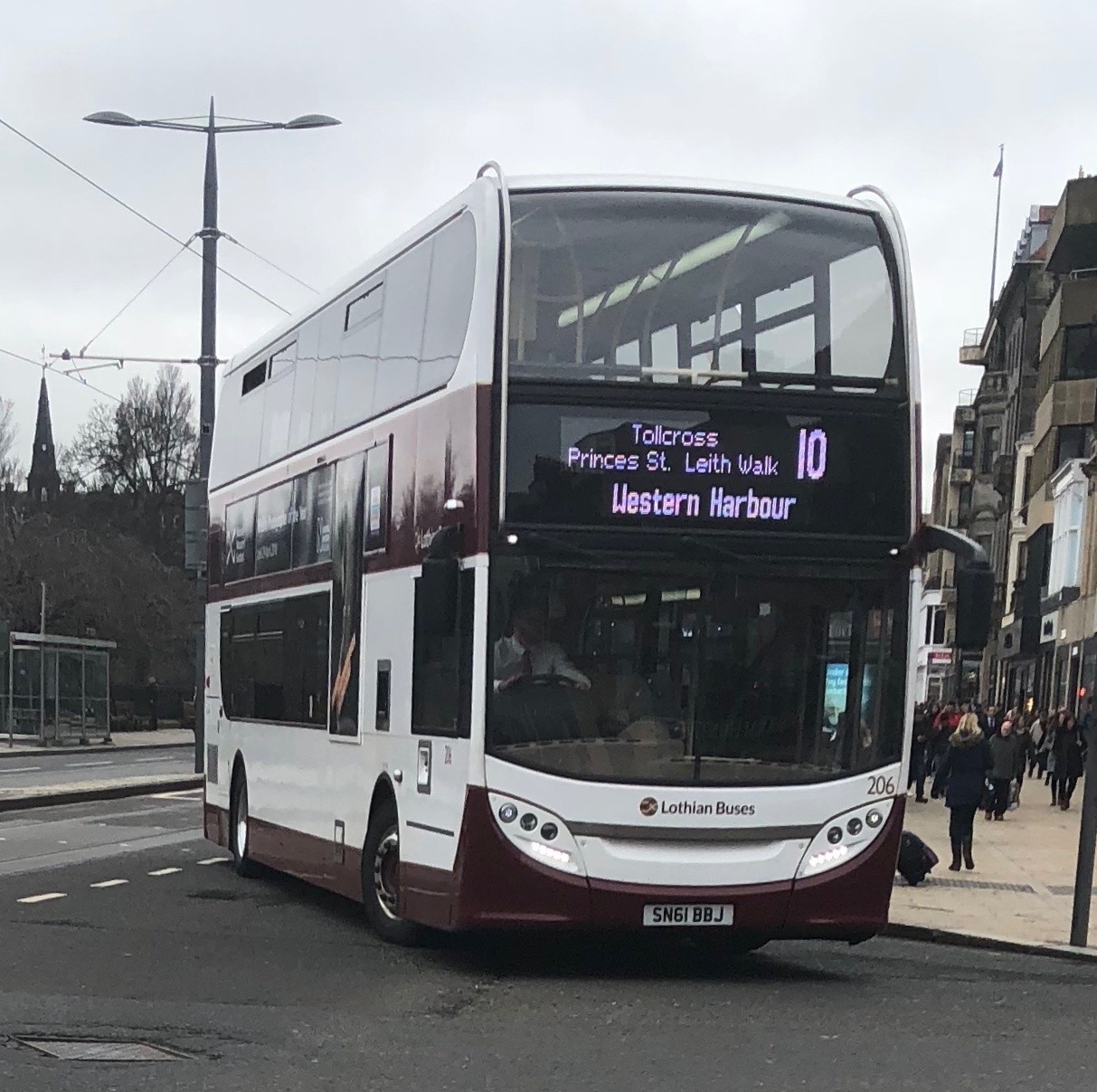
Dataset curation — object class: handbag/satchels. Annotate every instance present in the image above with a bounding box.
[1080,749,1088,764]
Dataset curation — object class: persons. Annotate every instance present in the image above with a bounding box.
[491,593,591,694]
[146,677,160,731]
[908,696,1093,821]
[930,713,994,871]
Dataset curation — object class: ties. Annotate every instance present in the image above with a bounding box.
[521,650,532,674]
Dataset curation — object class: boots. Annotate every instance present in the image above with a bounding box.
[962,847,974,870]
[948,841,962,872]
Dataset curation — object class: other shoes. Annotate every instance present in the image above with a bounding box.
[1057,793,1070,812]
[994,814,1004,821]
[915,796,928,803]
[984,812,992,820]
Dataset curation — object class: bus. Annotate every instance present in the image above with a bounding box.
[203,158,984,943]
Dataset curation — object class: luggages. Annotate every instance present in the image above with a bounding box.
[896,830,939,886]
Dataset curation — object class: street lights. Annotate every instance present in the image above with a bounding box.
[84,108,341,775]
